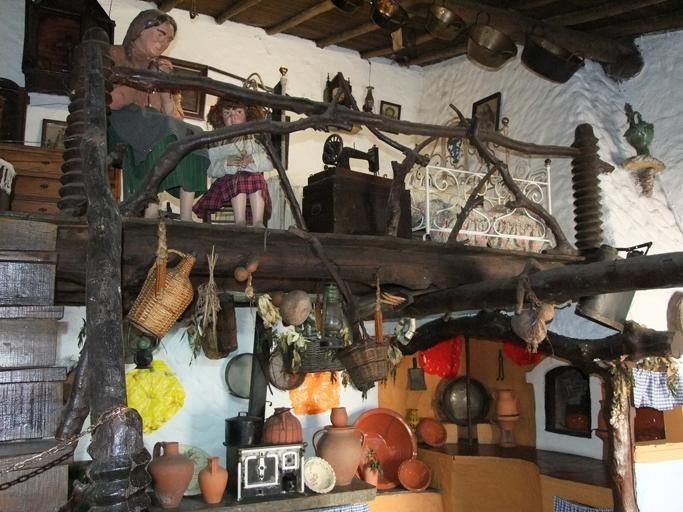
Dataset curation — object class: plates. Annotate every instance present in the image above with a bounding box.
[164,443,217,499]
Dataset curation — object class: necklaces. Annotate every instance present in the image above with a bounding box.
[233,135,249,155]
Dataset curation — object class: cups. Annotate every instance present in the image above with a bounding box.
[330,407,348,427]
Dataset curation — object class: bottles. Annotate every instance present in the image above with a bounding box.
[198,457,229,504]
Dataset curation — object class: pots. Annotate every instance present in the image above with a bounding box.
[326,0,585,85]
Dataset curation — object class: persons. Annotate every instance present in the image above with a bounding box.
[108,9,209,223]
[479,103,494,124]
[384,107,395,119]
[193,92,273,227]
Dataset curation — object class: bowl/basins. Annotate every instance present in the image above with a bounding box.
[347,408,419,491]
[398,458,431,494]
[438,375,491,424]
[224,410,263,447]
[419,418,448,447]
[300,455,338,494]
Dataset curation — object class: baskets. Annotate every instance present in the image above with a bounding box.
[127,249,196,339]
[295,338,388,389]
